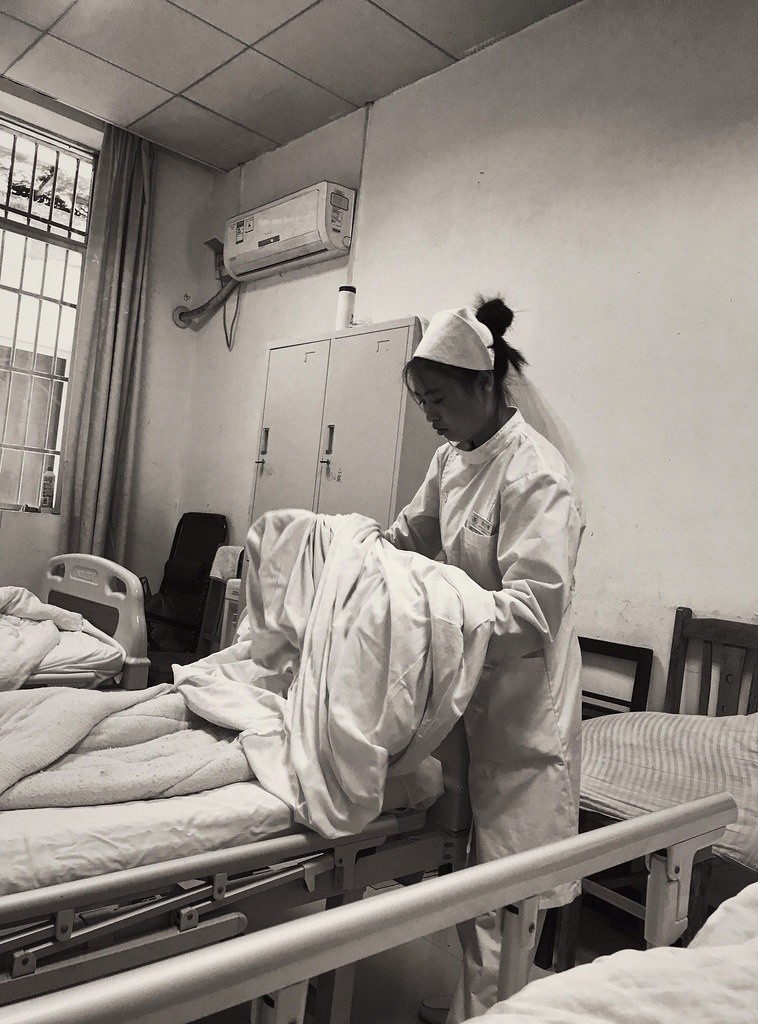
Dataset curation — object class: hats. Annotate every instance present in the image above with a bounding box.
[412,306,496,370]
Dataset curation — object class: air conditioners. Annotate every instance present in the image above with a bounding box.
[223,181,357,283]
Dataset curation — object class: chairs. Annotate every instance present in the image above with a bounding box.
[145,512,229,653]
[577,635,652,931]
[554,607,758,972]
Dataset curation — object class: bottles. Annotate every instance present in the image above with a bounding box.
[40,467,55,508]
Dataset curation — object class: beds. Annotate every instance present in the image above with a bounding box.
[0,577,483,1024]
[0,554,151,692]
[0,791,758,1024]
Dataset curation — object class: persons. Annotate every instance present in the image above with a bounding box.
[379,294,585,1024]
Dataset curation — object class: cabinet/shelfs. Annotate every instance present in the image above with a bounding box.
[246,316,445,562]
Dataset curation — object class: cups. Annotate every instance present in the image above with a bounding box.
[335,286,356,332]
